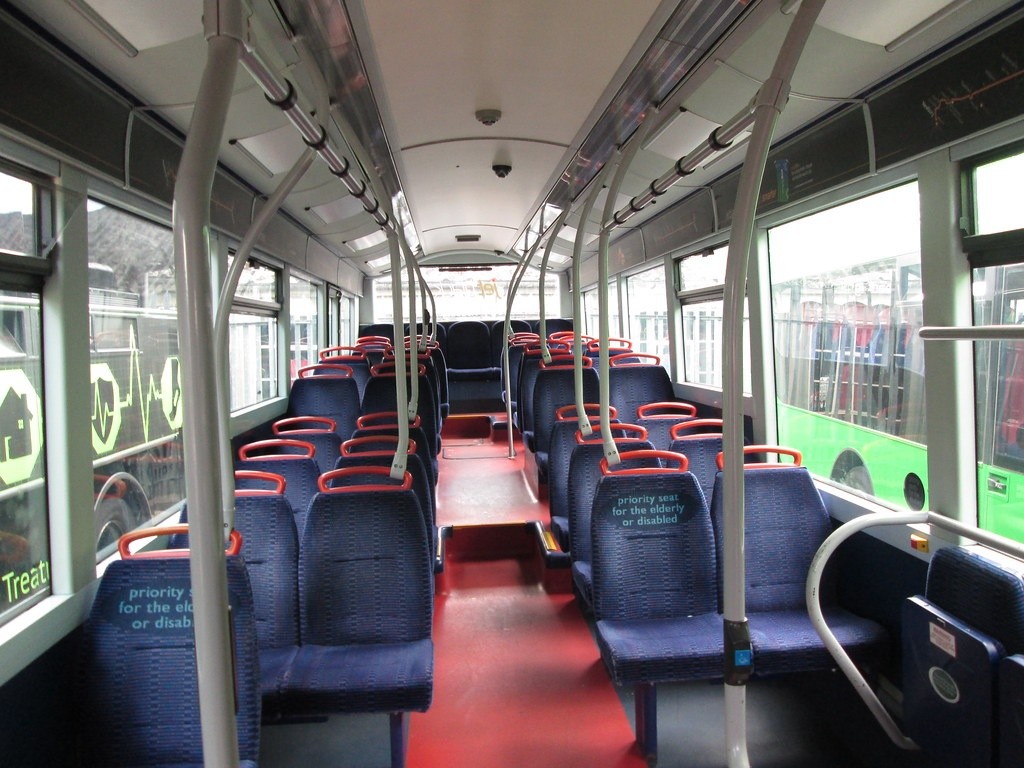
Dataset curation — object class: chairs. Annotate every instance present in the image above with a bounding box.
[75,523,260,768]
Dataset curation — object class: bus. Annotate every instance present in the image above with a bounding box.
[772,248,1024,541]
[0,250,186,631]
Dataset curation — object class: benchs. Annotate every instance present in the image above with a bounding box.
[360,318,573,412]
[169,335,453,767]
[500,330,891,768]
[900,547,1024,768]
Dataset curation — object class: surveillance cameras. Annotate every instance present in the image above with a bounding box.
[493,164,512,178]
[494,250,504,256]
